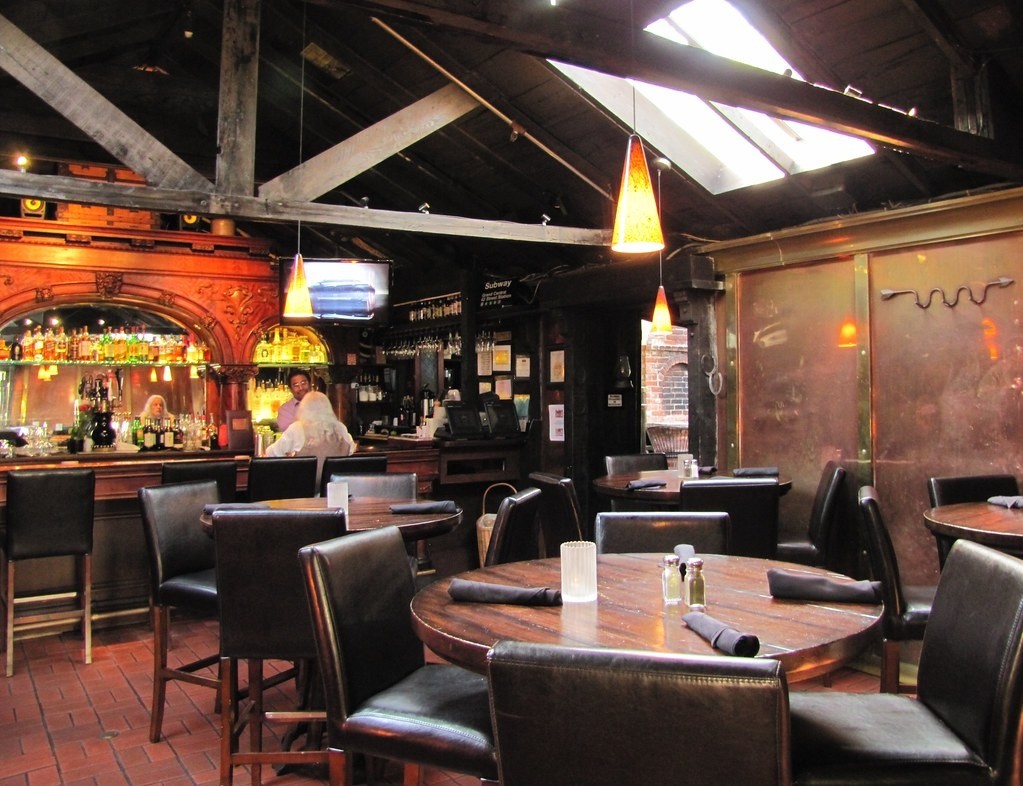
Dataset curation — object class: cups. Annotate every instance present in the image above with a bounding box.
[560,541,598,604]
[326,482,349,515]
[677,453,693,479]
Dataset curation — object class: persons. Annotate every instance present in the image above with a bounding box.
[276,369,311,432]
[139,394,176,427]
[266,390,357,498]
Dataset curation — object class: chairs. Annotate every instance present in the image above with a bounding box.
[140,454,1023,786]
[0,468,95,677]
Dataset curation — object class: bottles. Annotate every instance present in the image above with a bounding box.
[691,459,699,478]
[682,459,691,478]
[247,328,325,457]
[662,555,682,605]
[409,296,462,321]
[0,324,219,459]
[684,558,706,608]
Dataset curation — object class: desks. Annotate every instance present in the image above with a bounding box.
[409,551,888,681]
[196,498,461,543]
[594,471,795,500]
[921,502,1023,553]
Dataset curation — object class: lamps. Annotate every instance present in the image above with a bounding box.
[418,202,430,213]
[608,0,665,252]
[649,158,673,334]
[360,197,369,208]
[281,0,312,318]
[541,213,551,225]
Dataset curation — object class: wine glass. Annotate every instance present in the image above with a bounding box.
[382,332,496,359]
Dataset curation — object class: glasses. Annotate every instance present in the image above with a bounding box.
[293,381,306,387]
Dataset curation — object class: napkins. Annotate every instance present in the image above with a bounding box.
[768,568,883,604]
[202,503,268,511]
[733,467,779,477]
[625,480,666,491]
[391,501,460,515]
[445,577,562,605]
[682,611,760,657]
[987,496,1023,508]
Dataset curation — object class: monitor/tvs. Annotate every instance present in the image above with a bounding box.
[278,256,394,329]
[484,400,522,434]
[444,400,484,436]
